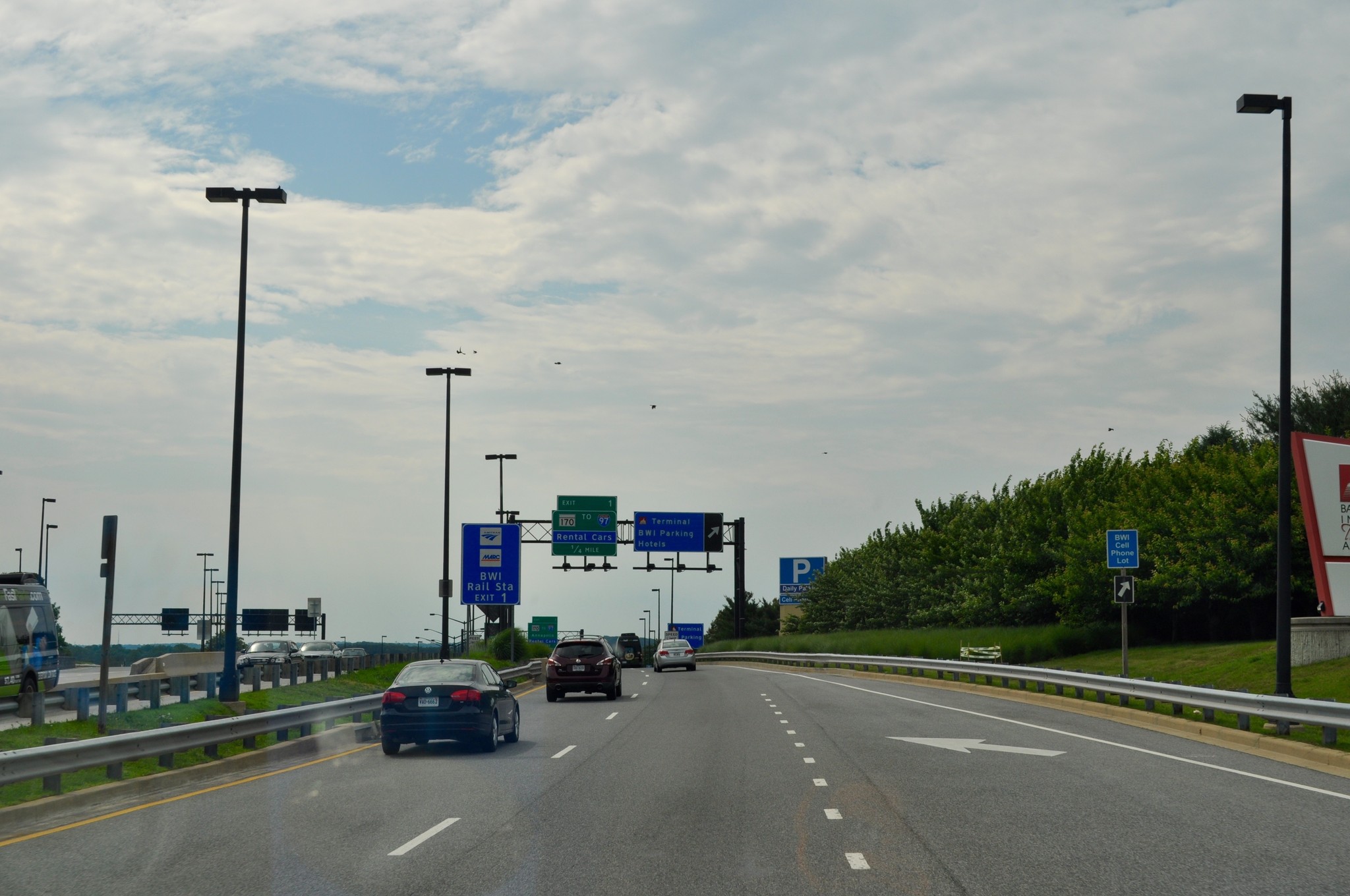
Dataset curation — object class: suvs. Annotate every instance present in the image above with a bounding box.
[546,634,622,702]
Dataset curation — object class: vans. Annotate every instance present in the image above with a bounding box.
[614,632,643,668]
[0,572,60,704]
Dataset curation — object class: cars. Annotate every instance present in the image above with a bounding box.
[297,640,343,659]
[653,638,696,673]
[380,658,520,755]
[341,648,369,657]
[237,639,299,674]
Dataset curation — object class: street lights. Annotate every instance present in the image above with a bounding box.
[485,454,520,627]
[382,635,387,655]
[425,367,472,659]
[341,636,346,649]
[639,558,674,668]
[196,552,227,652]
[15,548,22,572]
[38,494,58,590]
[1235,94,1302,725]
[205,186,288,701]
[416,613,487,661]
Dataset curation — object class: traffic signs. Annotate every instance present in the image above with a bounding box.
[461,522,521,605]
[550,495,617,557]
[633,511,724,552]
[527,615,557,649]
[668,623,704,649]
[779,556,825,606]
[1105,529,1139,568]
